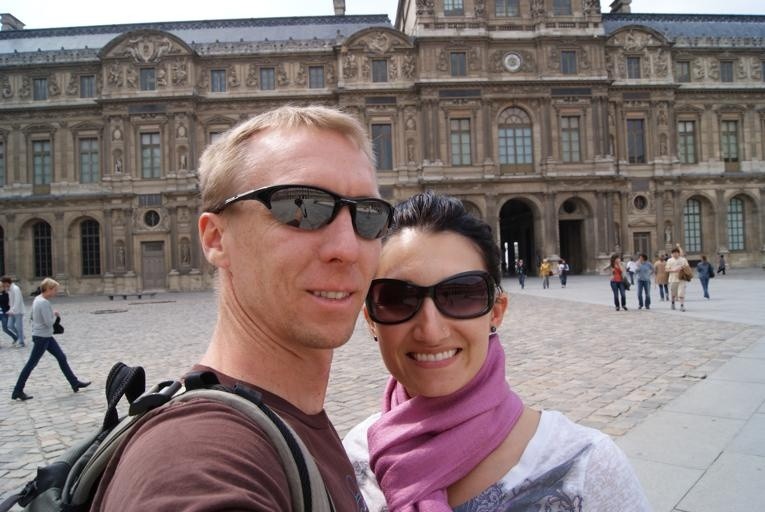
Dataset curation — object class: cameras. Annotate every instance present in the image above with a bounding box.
[295,199,302,205]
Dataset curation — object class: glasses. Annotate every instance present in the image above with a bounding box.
[205,182,393,242]
[361,269,498,324]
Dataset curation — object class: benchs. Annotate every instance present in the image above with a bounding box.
[97,291,156,301]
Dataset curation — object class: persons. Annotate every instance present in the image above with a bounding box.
[609,248,726,312]
[0,281,18,345]
[516,259,526,289]
[88,106,382,511]
[540,259,551,290]
[11,278,91,401]
[341,193,655,512]
[0,276,26,347]
[558,260,570,288]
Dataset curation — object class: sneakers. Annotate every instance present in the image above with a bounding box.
[16,343,25,348]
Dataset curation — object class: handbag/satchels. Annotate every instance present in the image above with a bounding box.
[708,269,715,279]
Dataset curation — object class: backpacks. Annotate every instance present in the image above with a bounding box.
[0,360,150,511]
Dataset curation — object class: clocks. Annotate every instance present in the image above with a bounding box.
[501,51,524,73]
